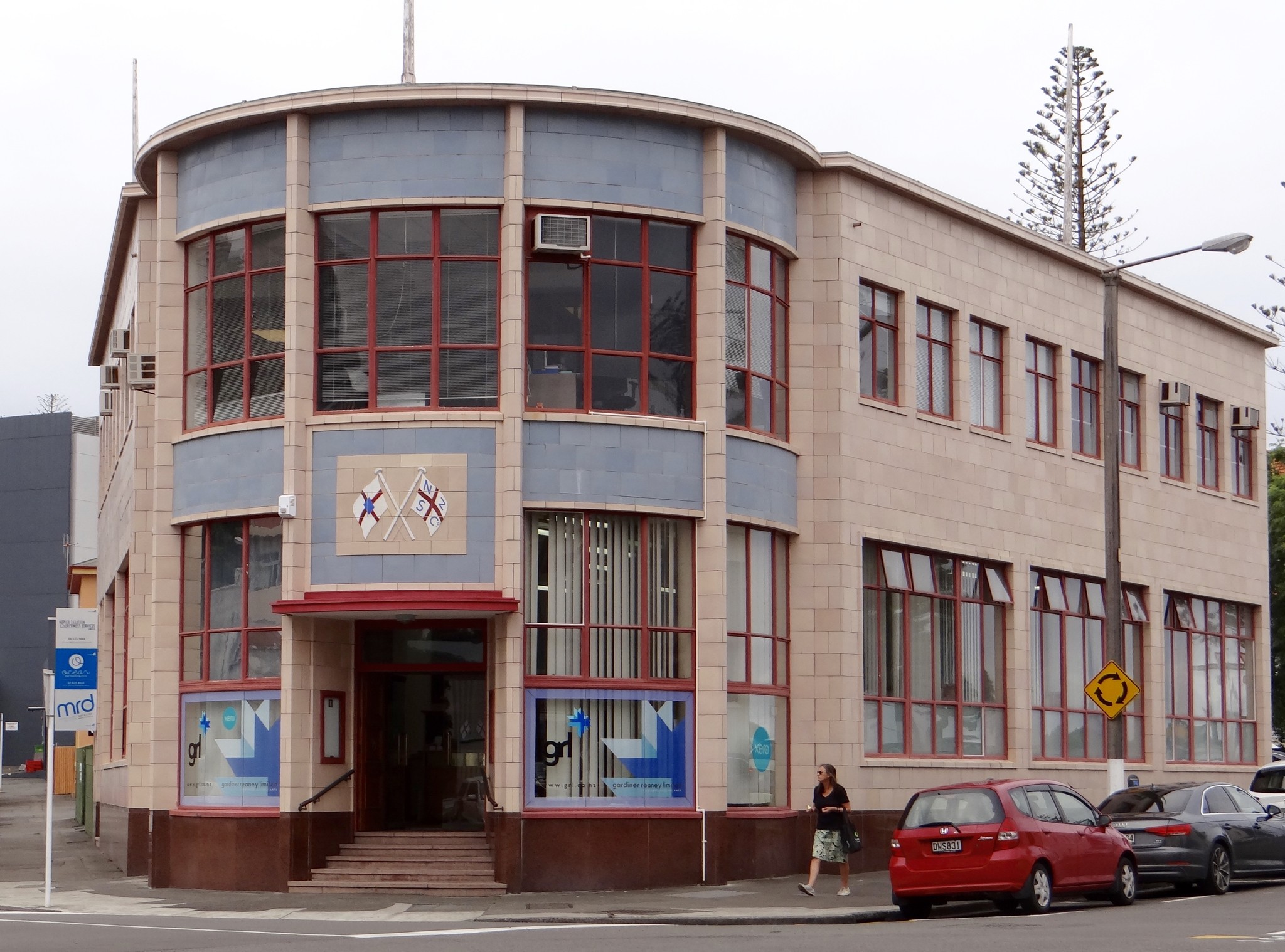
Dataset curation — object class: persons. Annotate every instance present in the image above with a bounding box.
[798,764,850,896]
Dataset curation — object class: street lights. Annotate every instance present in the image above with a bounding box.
[1104,226,1254,767]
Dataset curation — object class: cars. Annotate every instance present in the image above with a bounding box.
[888,778,1138,913]
[1098,782,1284,892]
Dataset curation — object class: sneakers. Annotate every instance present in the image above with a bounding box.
[798,883,815,896]
[837,886,851,896]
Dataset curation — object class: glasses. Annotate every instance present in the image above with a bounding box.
[817,771,823,774]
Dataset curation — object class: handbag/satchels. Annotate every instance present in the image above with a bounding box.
[840,808,862,853]
